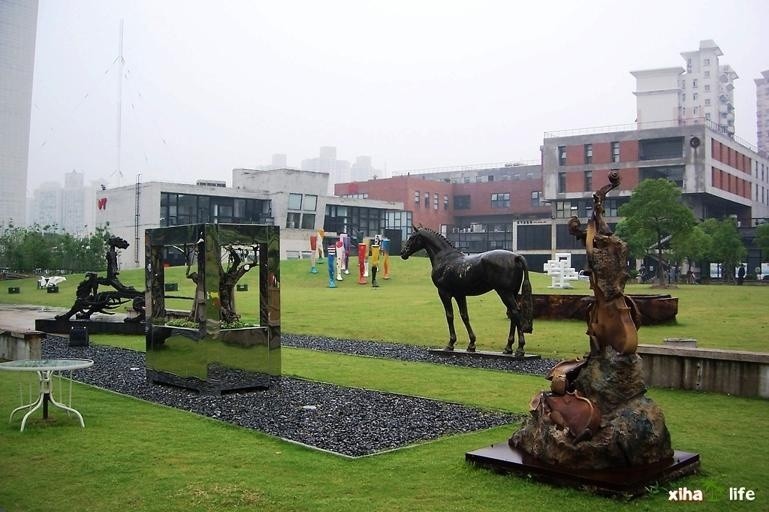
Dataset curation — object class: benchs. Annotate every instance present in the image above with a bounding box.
[636,344,769,399]
[0,325,47,360]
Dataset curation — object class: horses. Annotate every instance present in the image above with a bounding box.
[400,223,534,359]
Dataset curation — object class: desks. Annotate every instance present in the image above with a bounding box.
[0,358,95,432]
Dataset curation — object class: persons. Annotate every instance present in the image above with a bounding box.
[737,264,745,285]
[639,264,649,284]
